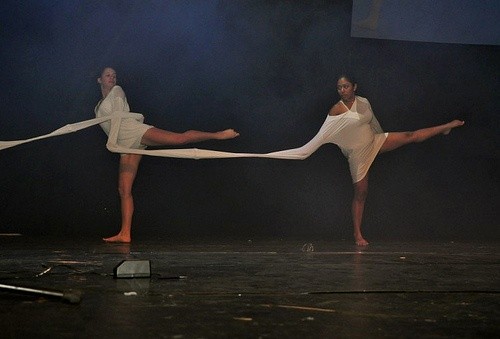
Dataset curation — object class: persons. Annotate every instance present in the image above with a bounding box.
[94,65,240,242]
[298,72,465,247]
